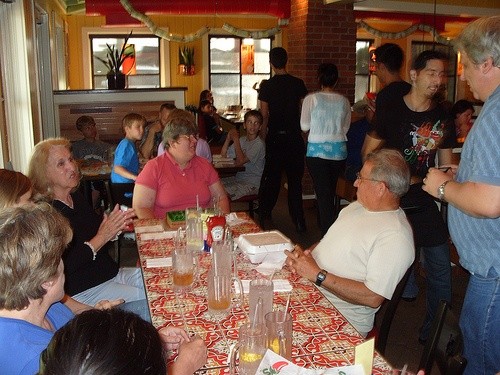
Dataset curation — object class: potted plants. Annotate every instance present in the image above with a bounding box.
[176,46,196,76]
[92,30,134,90]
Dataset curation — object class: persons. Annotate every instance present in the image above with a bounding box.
[454,99,474,140]
[350,41,412,192]
[67,115,113,167]
[138,102,228,160]
[199,90,234,146]
[300,62,352,238]
[26,136,140,298]
[0,199,209,375]
[35,307,172,375]
[258,47,307,237]
[283,149,416,341]
[220,110,266,201]
[132,118,231,219]
[0,168,154,324]
[360,49,465,347]
[422,13,500,375]
[110,113,146,211]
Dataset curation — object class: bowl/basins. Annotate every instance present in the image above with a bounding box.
[233,230,295,264]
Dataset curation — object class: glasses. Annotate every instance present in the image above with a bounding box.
[356,171,385,183]
[176,134,197,141]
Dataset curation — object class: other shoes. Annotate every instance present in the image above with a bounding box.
[421,339,467,372]
[296,222,307,232]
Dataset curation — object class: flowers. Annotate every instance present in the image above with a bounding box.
[367,92,376,104]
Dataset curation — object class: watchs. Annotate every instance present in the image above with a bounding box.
[316,269,327,287]
[438,180,456,200]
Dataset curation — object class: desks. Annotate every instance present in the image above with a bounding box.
[133,211,396,375]
[80,153,246,265]
[218,109,247,138]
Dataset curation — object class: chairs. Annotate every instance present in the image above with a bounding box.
[108,178,135,265]
[415,299,470,375]
[371,251,420,355]
[240,195,258,221]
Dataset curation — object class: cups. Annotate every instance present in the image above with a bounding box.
[249,278,274,325]
[264,311,292,362]
[171,197,243,315]
[229,322,268,375]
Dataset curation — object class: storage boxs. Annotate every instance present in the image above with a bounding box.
[237,229,295,263]
